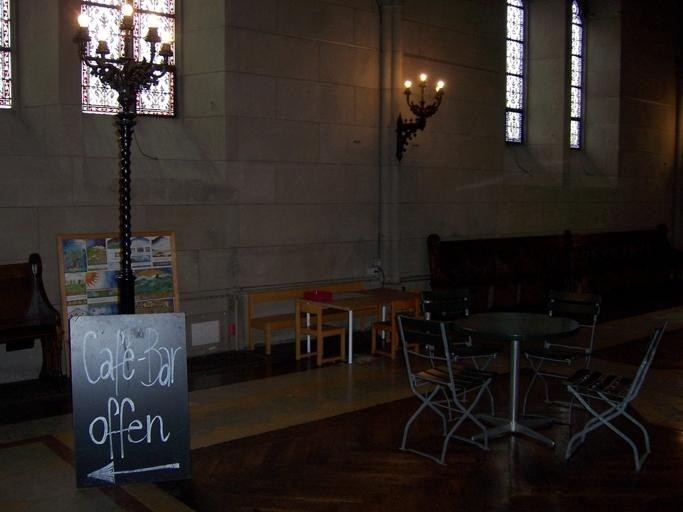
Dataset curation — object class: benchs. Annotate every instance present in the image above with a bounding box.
[426,220,681,320]
[0,252,61,385]
[249,283,364,355]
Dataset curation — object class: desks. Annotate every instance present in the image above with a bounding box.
[302,290,413,363]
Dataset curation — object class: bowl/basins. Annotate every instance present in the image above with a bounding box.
[302,291,334,302]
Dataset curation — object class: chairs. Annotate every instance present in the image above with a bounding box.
[565,323,667,472]
[372,296,621,472]
[295,302,345,366]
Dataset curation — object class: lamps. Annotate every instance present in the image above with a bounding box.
[393,67,448,158]
[71,4,176,315]
